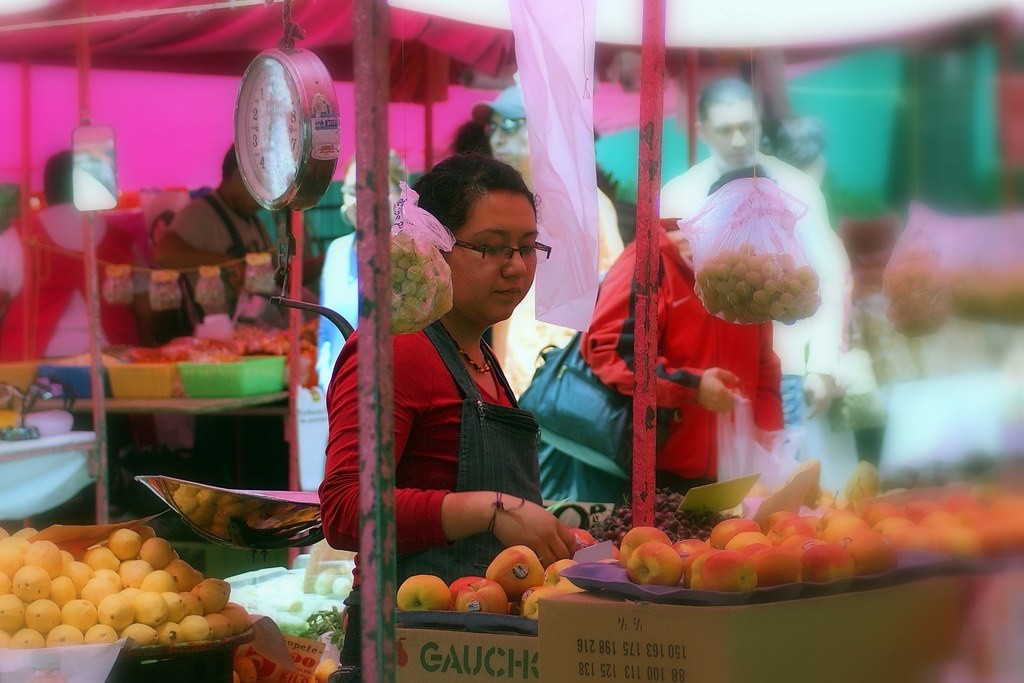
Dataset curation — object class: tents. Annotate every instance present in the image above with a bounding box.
[0,0,1022,575]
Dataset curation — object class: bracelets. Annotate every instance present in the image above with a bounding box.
[487,489,527,537]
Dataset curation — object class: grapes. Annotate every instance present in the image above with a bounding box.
[384,229,452,333]
[697,245,821,326]
[883,259,971,330]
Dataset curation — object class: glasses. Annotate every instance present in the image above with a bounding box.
[454,240,552,265]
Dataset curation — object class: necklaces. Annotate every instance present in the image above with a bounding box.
[438,320,490,376]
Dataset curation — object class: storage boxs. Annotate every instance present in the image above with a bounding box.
[0,353,1018,682]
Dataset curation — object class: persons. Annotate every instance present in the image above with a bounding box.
[148,137,325,497]
[310,153,576,683]
[1,147,153,520]
[656,76,850,423]
[584,162,783,496]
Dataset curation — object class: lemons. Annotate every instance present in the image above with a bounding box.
[0,525,252,648]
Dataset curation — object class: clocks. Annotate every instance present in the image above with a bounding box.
[233,44,342,213]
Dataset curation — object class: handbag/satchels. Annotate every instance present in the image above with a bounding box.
[390,181,456,335]
[882,199,1024,337]
[677,178,822,327]
[517,332,684,480]
[716,387,813,499]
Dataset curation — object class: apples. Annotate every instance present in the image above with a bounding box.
[395,492,1016,619]
